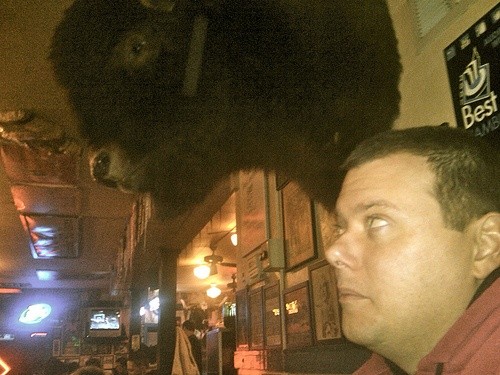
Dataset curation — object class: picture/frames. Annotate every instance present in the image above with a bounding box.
[261,278,285,351]
[275,167,292,191]
[248,286,265,351]
[235,287,250,352]
[307,258,347,346]
[280,176,318,272]
[50,338,129,370]
[282,280,314,351]
[236,167,270,258]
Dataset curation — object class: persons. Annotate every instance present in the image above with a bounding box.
[43,357,128,375]
[183,320,202,375]
[325,127,499,375]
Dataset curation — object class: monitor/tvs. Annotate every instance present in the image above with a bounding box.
[88,307,122,337]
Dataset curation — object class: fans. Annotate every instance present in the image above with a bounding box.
[226,273,237,289]
[203,243,238,275]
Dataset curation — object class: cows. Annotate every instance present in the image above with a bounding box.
[46,0,403,221]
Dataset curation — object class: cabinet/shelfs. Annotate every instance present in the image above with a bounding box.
[205,327,236,375]
